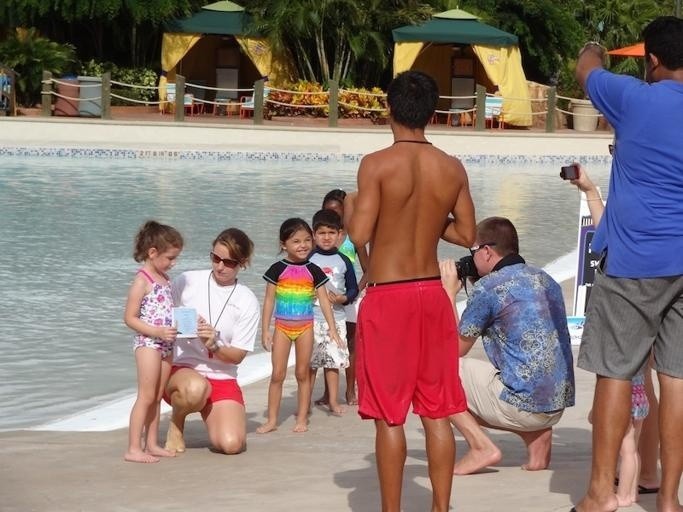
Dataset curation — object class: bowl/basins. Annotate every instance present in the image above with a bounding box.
[393,137,433,145]
[206,271,238,328]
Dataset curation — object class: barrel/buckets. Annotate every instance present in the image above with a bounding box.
[614,477,661,494]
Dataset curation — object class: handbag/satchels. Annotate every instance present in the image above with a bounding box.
[204,331,222,353]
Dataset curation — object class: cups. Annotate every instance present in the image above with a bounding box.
[469,243,497,256]
[210,252,238,268]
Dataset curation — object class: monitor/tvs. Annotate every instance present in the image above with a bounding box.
[168,304,198,338]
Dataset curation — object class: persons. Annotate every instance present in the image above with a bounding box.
[123,219,184,465]
[568,140,659,497]
[299,209,360,414]
[309,188,367,419]
[340,69,477,512]
[255,216,346,435]
[573,12,681,512]
[439,215,577,476]
[162,227,261,455]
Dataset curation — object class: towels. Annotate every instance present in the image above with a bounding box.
[77,76,103,117]
[54,79,80,117]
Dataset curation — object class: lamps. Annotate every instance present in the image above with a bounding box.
[239,88,271,119]
[473,97,504,129]
[163,83,195,117]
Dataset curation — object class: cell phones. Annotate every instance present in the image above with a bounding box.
[559,165,579,181]
[456,255,479,278]
